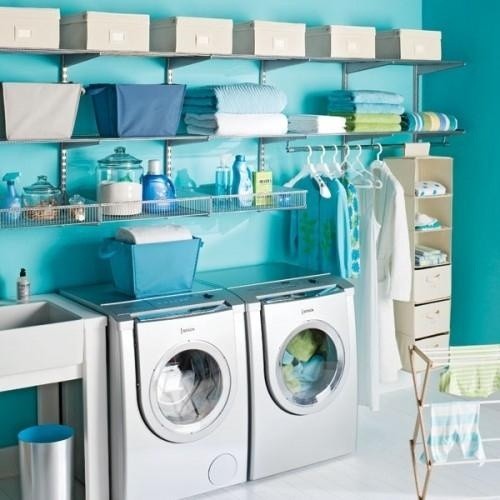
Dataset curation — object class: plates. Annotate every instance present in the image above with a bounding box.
[0,44,465,144]
[383,156,453,374]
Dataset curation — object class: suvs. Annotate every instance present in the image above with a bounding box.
[234,154,253,208]
[140,158,177,212]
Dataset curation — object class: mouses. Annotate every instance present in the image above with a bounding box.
[283,143,385,199]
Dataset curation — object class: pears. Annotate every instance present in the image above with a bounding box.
[214,155,232,208]
[2,171,24,222]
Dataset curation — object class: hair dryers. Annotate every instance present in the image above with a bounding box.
[58,268,250,496]
[196,261,356,481]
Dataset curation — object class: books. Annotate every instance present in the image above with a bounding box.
[96,236,203,299]
[86,84,188,137]
[2,82,85,141]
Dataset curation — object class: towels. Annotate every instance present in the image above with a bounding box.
[17,423,76,500]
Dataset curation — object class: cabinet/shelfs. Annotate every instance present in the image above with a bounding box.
[215,159,231,209]
[68,195,86,227]
[23,174,61,221]
[95,145,145,217]
[231,152,255,206]
[15,267,32,304]
